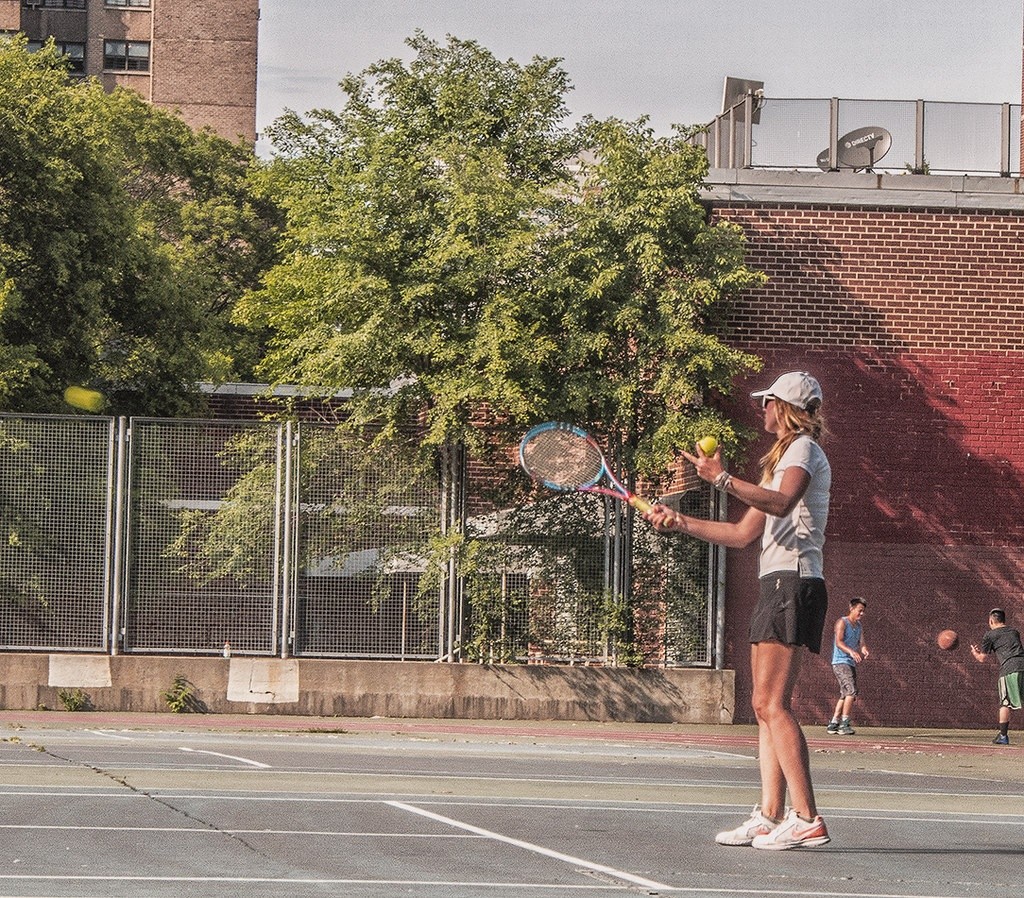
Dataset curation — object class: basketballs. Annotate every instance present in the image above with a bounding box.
[938,630,959,650]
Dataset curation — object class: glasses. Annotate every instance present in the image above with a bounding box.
[762,394,775,407]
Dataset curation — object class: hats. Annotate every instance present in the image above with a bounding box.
[752,372,822,412]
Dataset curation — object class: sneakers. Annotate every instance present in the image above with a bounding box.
[716,806,789,845]
[992,734,1008,744]
[827,723,839,734]
[839,724,855,735]
[752,811,831,851]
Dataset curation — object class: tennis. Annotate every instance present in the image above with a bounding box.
[698,436,718,456]
[64,385,105,412]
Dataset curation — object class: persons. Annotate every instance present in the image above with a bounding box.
[641,372,831,851]
[970,609,1024,745]
[828,598,869,736]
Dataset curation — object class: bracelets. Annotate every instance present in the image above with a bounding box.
[675,513,680,531]
[714,471,732,492]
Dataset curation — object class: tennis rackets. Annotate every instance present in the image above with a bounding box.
[519,422,675,530]
